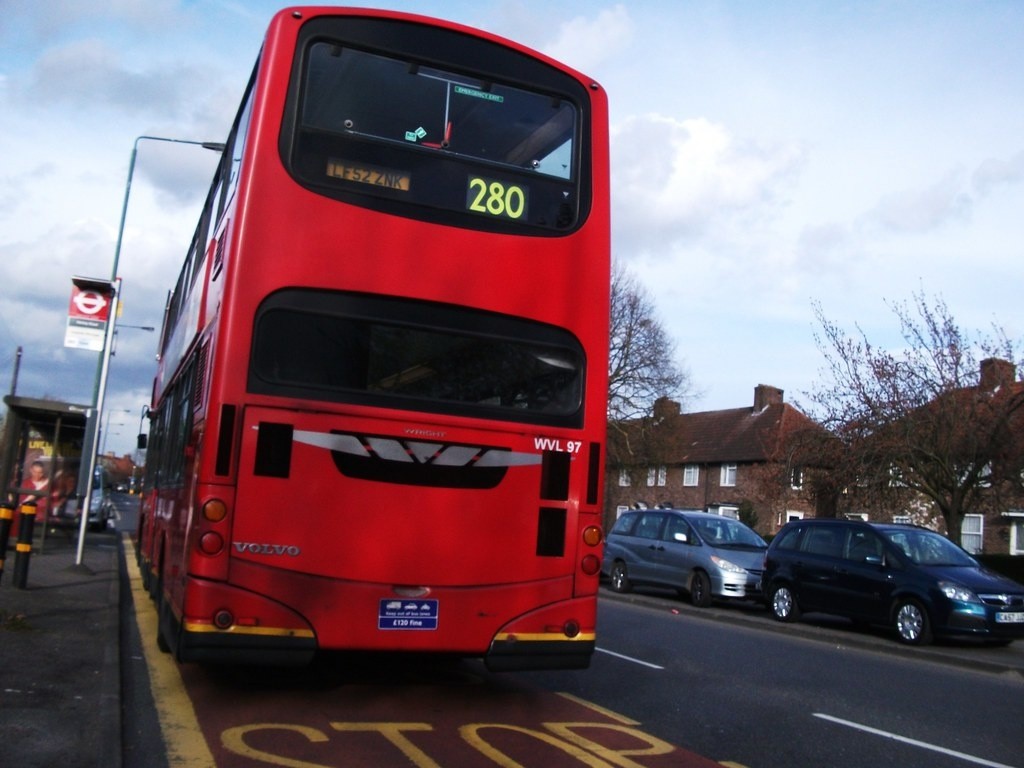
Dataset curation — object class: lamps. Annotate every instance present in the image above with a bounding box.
[1000,528,1009,542]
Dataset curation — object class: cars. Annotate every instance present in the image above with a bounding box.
[760,517,1024,646]
[115,484,127,491]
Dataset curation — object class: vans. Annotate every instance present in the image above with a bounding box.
[606,512,771,607]
[69,464,108,528]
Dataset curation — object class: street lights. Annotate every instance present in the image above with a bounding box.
[72,136,228,563]
[100,406,129,464]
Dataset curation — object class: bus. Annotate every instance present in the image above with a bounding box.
[136,3,612,660]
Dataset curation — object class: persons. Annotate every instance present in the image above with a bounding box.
[17,436,81,518]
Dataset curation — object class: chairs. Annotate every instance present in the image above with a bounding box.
[886,543,905,570]
[671,524,687,540]
[641,521,658,538]
[812,535,830,554]
[850,537,874,560]
[700,527,718,541]
